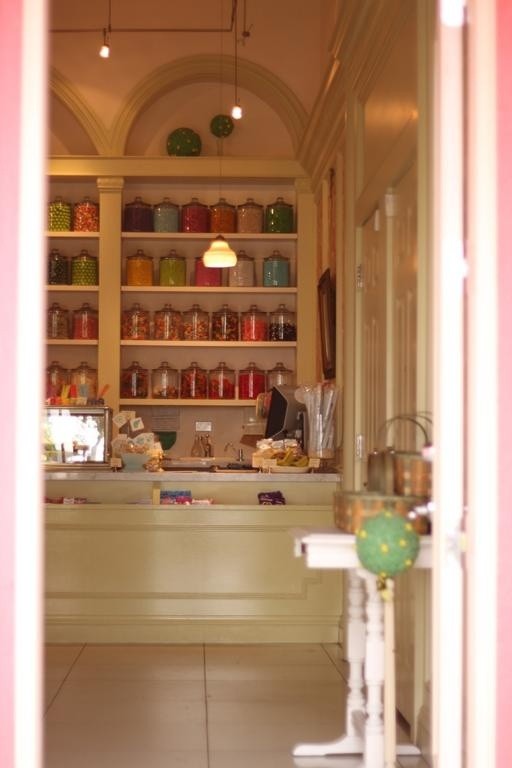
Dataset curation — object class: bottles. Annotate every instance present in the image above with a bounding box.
[48,247,292,287]
[47,193,297,234]
[47,302,296,341]
[44,360,295,407]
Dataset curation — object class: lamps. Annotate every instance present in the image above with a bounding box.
[204,53,238,268]
[231,59,244,121]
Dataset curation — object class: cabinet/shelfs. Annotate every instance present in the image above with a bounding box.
[121,177,318,467]
[47,174,100,399]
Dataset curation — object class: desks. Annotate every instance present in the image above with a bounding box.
[287,525,432,768]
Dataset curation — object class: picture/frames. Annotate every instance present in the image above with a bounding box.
[47,406,111,468]
